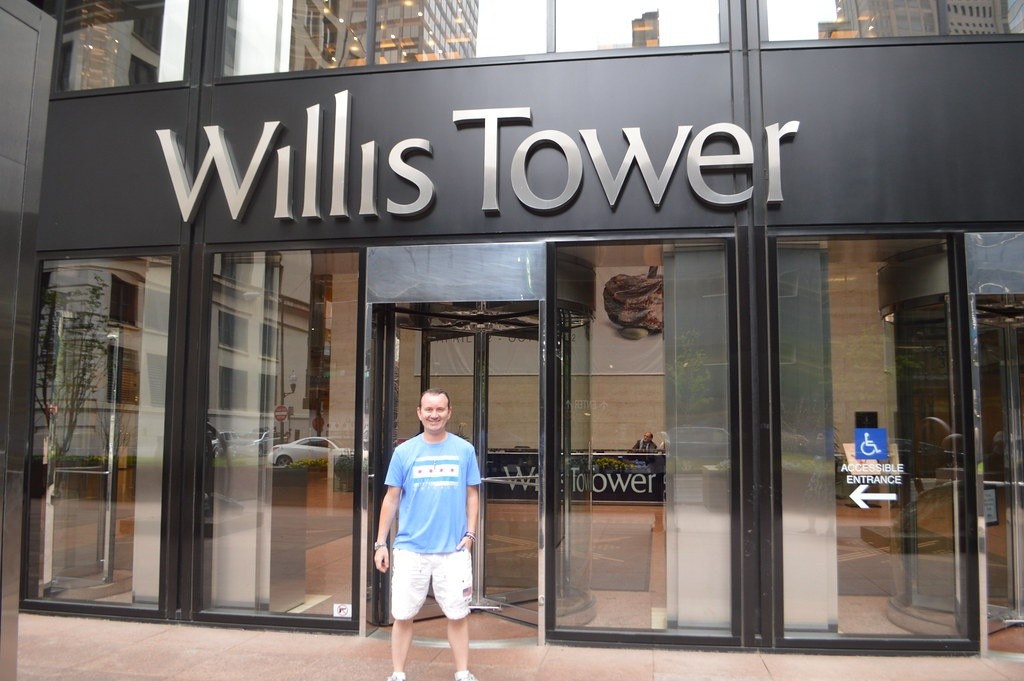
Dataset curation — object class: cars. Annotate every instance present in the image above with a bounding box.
[207,421,225,458]
[241,431,287,457]
[220,431,240,460]
[268,437,354,467]
[667,426,729,473]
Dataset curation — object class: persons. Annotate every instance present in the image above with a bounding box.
[631,433,657,452]
[374,386,482,681]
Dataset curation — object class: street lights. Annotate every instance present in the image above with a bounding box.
[242,292,297,446]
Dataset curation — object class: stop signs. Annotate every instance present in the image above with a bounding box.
[274,406,288,422]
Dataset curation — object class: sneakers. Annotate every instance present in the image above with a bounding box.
[387,672,407,681]
[454,671,478,681]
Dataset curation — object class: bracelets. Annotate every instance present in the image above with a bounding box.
[464,531,477,543]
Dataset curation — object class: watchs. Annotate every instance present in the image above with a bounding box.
[373,541,387,550]
